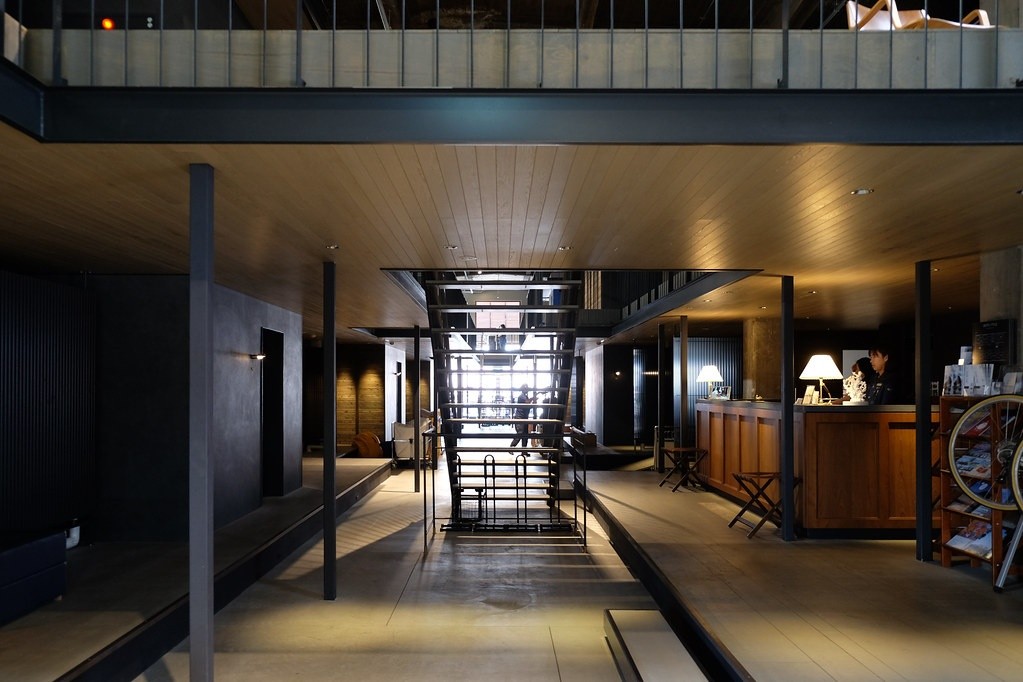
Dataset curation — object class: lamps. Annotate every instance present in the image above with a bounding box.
[251,355,266,360]
[799,355,844,404]
[695,365,723,400]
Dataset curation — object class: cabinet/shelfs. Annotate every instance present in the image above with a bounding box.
[938,395,1023,585]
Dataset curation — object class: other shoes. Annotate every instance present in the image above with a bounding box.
[522,452,530,456]
[509,452,513,455]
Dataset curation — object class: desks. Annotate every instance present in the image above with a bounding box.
[727,472,799,539]
[659,447,707,492]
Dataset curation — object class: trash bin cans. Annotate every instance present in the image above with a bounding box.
[394,417,430,458]
[654,426,675,469]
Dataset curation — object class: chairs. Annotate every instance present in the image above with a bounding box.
[846,0,1006,31]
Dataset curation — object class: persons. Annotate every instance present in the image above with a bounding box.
[509,384,533,456]
[855,344,897,404]
